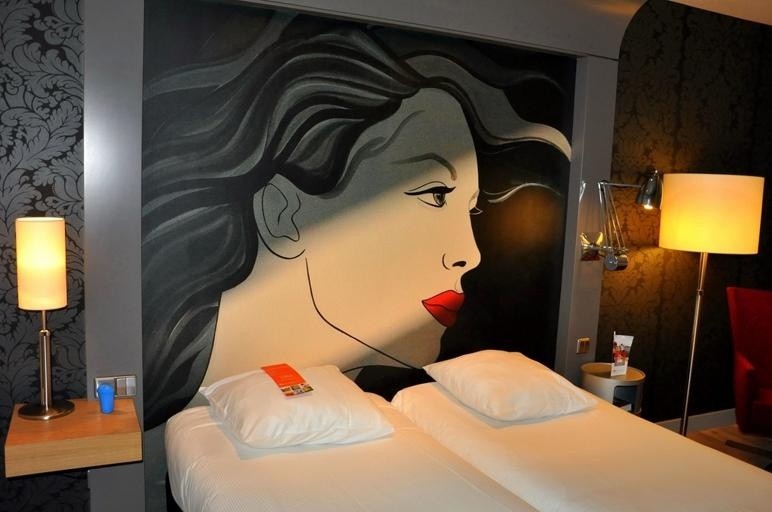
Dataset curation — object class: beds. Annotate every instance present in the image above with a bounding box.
[397,378,770,511]
[162,378,522,512]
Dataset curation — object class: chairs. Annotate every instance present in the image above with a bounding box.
[725,284,772,456]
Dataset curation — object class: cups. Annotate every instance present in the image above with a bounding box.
[97,385,115,413]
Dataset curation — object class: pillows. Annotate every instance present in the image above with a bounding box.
[421,346,598,423]
[198,363,396,451]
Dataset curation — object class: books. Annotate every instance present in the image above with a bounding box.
[259,362,314,400]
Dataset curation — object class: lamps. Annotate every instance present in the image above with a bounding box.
[579,165,766,436]
[13,216,74,421]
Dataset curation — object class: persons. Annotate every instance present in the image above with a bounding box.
[141,29,574,437]
